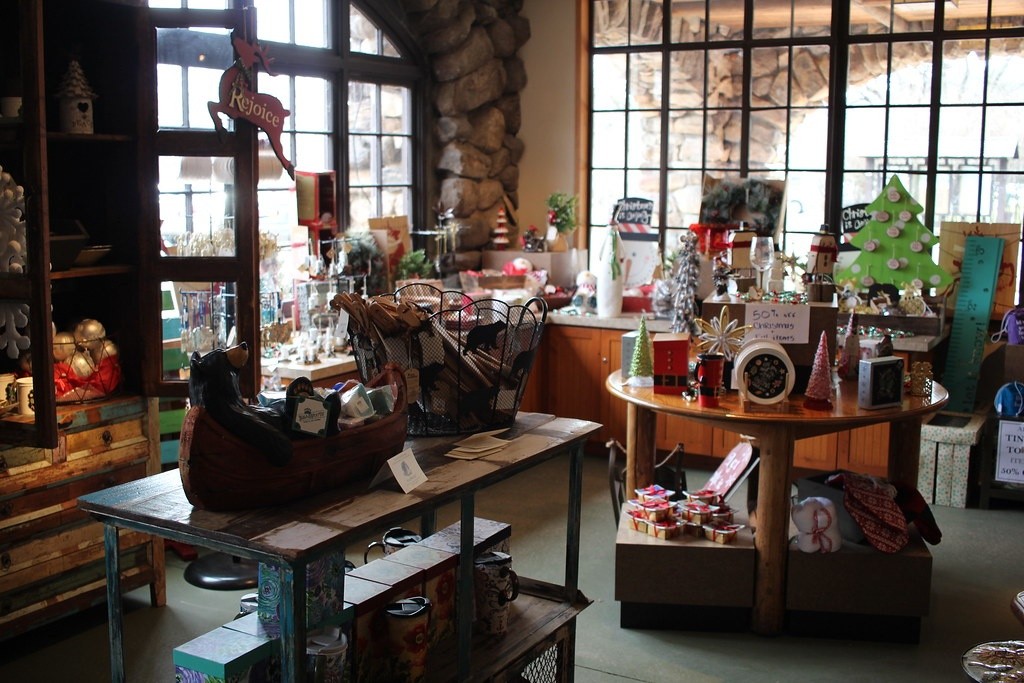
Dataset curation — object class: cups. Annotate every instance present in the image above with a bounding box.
[364,526,421,565]
[0,373,15,404]
[16,377,34,416]
[1,97,22,117]
[306,626,348,683]
[474,551,521,636]
[693,352,725,408]
[386,596,432,682]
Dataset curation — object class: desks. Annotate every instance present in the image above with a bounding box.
[76,411,603,683]
[606,365,949,636]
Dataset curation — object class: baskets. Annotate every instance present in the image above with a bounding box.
[346,282,548,435]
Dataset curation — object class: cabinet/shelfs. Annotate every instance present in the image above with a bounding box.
[261,323,915,485]
[0,0,262,645]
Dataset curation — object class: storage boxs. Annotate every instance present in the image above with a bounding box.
[615,501,756,608]
[702,288,839,365]
[483,247,578,290]
[839,296,947,336]
[787,515,932,616]
[916,408,987,509]
[172,516,512,683]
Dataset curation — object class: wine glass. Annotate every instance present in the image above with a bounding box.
[749,237,775,289]
